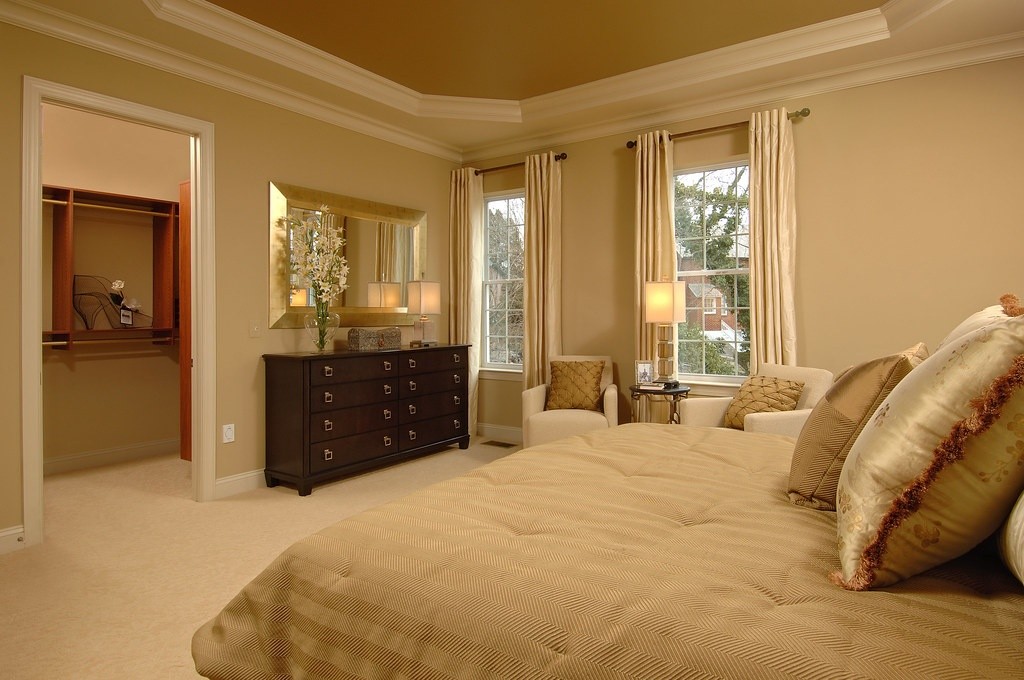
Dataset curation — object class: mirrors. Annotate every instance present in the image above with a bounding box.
[268,180,429,330]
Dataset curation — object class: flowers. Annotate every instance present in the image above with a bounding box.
[111,279,141,312]
[276,203,350,346]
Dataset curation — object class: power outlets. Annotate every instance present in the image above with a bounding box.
[222,423,235,443]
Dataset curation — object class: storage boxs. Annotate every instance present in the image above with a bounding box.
[347,327,401,352]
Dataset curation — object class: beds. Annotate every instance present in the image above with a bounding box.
[191,421,1023,679]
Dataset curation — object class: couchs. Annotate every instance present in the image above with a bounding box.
[678,362,833,438]
[522,355,618,449]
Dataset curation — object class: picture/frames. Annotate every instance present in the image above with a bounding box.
[635,359,655,386]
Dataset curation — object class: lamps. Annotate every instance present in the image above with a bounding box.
[290,289,307,307]
[645,280,686,390]
[406,280,442,348]
[368,281,401,307]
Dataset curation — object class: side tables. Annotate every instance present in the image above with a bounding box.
[629,383,691,425]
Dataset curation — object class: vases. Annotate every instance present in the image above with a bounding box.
[303,312,340,354]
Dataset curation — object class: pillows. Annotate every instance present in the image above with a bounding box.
[981,490,1024,592]
[544,360,605,413]
[784,341,929,510]
[835,293,1024,591]
[724,375,806,430]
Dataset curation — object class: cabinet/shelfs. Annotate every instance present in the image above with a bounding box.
[41,183,179,353]
[262,343,473,497]
